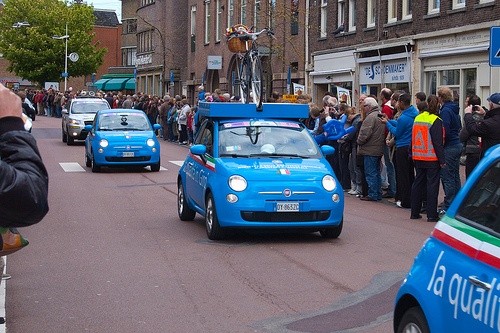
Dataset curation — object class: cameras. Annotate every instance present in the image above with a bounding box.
[472,106,478,111]
[378,114,383,118]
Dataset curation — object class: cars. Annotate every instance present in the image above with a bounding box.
[61,97,113,146]
[391,142,500,332]
[177,97,345,241]
[84,109,163,173]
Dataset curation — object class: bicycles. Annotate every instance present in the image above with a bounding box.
[226,25,275,108]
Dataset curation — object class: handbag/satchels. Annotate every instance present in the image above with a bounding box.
[0,227,30,256]
[465,144,481,157]
[340,141,352,160]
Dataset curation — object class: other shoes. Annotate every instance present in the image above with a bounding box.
[382,188,388,191]
[393,198,402,208]
[382,194,392,198]
[421,207,427,214]
[437,209,446,214]
[410,214,422,219]
[2,274,11,280]
[356,193,364,197]
[360,196,374,201]
[427,217,440,222]
[348,189,355,194]
[343,189,349,191]
[350,190,360,194]
[156,133,193,147]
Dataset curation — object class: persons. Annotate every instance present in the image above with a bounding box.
[178,99,190,145]
[436,86,464,214]
[0,82,49,260]
[378,92,419,208]
[355,97,386,201]
[274,130,328,155]
[410,94,446,221]
[12,80,500,214]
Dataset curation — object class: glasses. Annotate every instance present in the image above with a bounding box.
[364,104,371,108]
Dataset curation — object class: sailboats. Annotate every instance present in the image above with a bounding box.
[52,21,70,96]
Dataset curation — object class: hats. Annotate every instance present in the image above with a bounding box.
[322,95,338,108]
[487,92,500,104]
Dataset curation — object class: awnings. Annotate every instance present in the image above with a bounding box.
[92,78,136,91]
